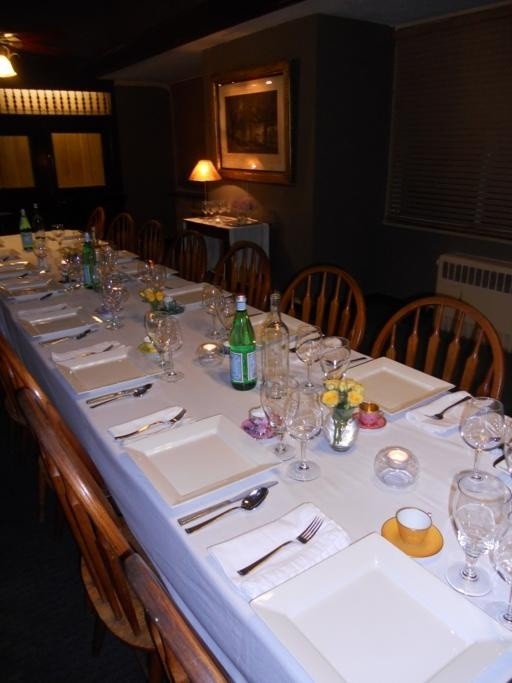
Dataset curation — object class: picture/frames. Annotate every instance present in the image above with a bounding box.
[209,60,292,186]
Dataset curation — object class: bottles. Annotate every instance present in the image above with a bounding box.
[228,293,258,390]
[260,293,290,396]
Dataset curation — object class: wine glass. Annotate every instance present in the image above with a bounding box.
[1,199,239,346]
[145,309,188,385]
[286,389,323,480]
[318,336,351,378]
[444,394,512,626]
[258,374,300,462]
[296,324,322,392]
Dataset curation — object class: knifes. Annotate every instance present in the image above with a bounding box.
[177,479,280,527]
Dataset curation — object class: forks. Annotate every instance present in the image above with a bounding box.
[290,335,323,355]
[56,344,115,366]
[109,404,189,443]
[235,513,330,575]
[426,393,472,422]
[86,382,159,404]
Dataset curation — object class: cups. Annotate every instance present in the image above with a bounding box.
[398,508,432,544]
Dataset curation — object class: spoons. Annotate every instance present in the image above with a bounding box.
[183,485,272,537]
[90,389,147,411]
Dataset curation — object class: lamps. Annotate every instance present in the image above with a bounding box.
[189,155,222,213]
[0,26,24,82]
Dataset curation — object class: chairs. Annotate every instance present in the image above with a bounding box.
[18,384,178,653]
[277,263,367,353]
[211,238,272,313]
[2,332,119,526]
[136,218,168,266]
[117,547,230,683]
[83,204,105,242]
[370,292,505,408]
[107,212,136,253]
[169,228,208,285]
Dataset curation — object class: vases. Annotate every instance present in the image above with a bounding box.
[324,410,361,452]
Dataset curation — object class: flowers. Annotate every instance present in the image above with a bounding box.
[137,287,164,304]
[322,376,365,410]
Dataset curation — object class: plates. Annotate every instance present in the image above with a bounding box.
[380,516,445,559]
[125,413,282,507]
[342,355,458,415]
[250,530,511,680]
[250,310,315,344]
[56,343,165,397]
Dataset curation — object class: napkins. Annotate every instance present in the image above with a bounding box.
[276,330,344,364]
[17,302,84,325]
[50,339,132,377]
[203,500,352,605]
[0,274,55,292]
[109,403,196,453]
[405,388,496,440]
